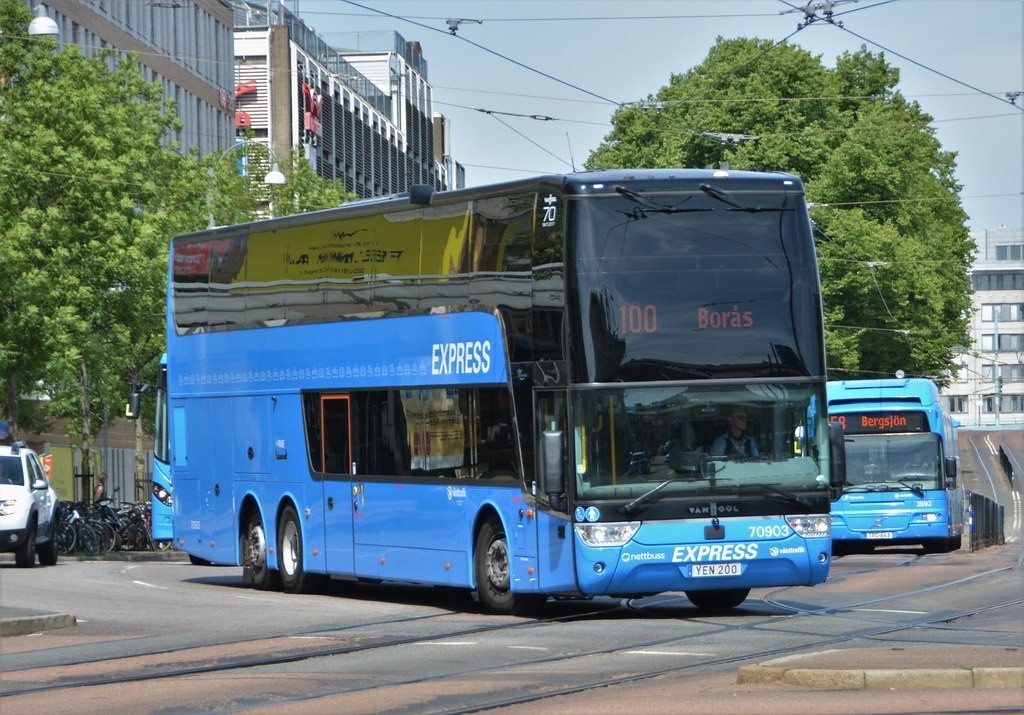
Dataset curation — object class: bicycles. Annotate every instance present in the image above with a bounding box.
[55,486,172,555]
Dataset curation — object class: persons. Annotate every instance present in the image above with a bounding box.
[709,405,760,461]
[92,472,108,502]
[903,451,929,470]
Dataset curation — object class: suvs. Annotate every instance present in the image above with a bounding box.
[0,440,61,568]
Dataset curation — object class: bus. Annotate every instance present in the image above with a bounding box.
[124,352,175,544]
[170,157,846,618]
[805,376,963,556]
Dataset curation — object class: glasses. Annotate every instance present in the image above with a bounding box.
[731,414,748,421]
